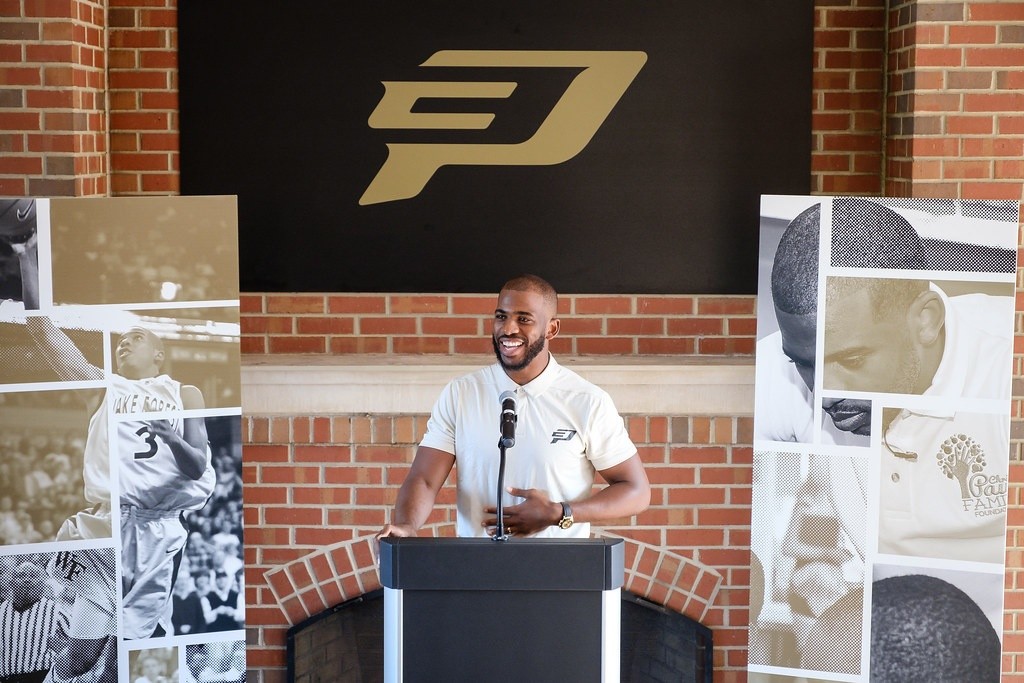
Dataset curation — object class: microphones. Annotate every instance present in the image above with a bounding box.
[499,390,519,447]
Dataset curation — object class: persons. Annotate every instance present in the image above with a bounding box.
[0,211,247,683]
[372,274,651,568]
[754,201,1016,683]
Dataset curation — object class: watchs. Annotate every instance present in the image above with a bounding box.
[558,501,574,529]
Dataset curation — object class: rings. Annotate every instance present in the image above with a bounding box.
[508,526,512,534]
